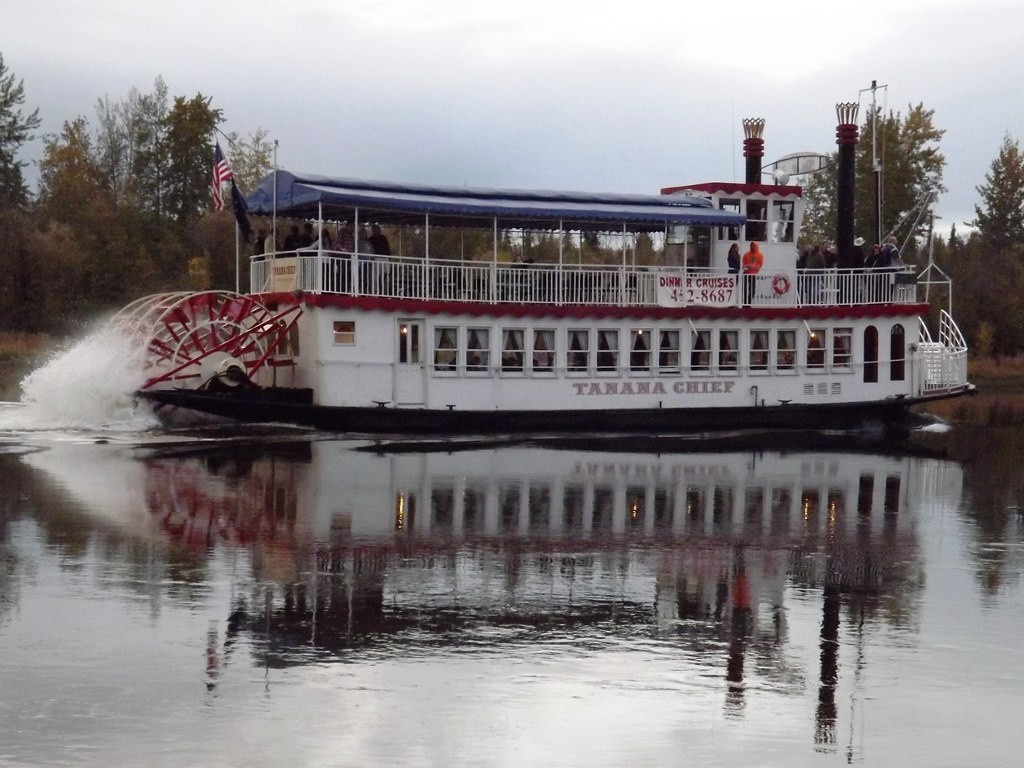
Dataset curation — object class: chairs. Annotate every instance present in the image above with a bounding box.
[450,266,638,303]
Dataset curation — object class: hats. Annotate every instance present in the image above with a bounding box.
[853,236,865,247]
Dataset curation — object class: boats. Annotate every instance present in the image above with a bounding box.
[95,77,978,435]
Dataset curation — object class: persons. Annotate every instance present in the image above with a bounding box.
[250,220,649,308]
[853,236,906,307]
[742,240,763,307]
[817,241,837,289]
[796,244,814,304]
[727,241,741,284]
[805,245,827,304]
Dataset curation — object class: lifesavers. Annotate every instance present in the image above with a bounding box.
[774,276,789,293]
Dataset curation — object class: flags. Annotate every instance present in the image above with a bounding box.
[211,143,234,210]
[231,182,252,245]
